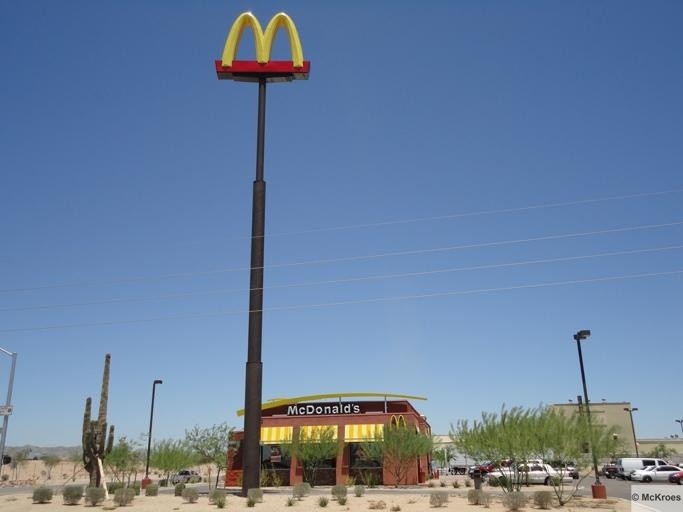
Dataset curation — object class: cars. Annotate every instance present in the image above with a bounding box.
[146,380,162,478]
[169,469,202,486]
[630,456,683,484]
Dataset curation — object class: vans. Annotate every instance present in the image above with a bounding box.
[604,458,668,481]
[468,456,579,486]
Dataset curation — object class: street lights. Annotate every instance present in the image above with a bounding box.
[606,428,617,458]
[624,407,640,458]
[573,330,601,484]
[676,419,683,432]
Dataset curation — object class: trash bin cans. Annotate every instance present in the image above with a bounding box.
[473,468,481,490]
